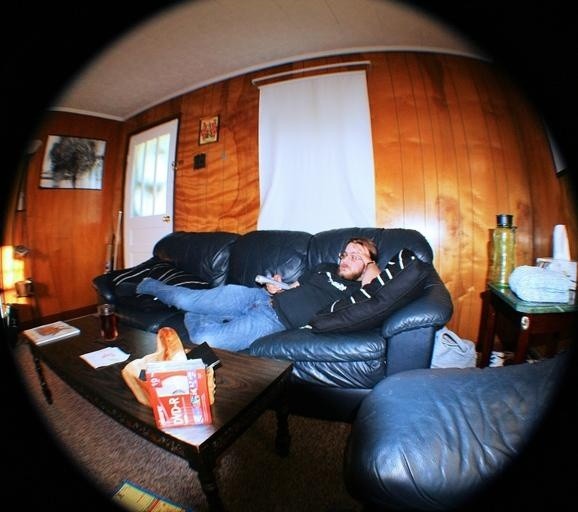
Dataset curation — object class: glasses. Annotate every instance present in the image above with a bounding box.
[338,252,364,262]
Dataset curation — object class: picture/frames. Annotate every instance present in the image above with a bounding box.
[198,114,220,145]
[39,134,108,191]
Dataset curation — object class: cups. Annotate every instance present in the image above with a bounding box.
[96,302,118,343]
[14,280,32,296]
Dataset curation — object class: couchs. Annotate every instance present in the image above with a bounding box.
[346,347,577,512]
[91,227,453,425]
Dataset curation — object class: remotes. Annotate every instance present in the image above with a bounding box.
[254,275,289,290]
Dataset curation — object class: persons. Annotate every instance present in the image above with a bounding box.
[114,238,381,350]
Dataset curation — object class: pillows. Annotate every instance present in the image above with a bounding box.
[310,248,432,335]
[111,263,212,314]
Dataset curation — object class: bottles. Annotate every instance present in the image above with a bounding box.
[493,217,517,288]
[98,304,117,342]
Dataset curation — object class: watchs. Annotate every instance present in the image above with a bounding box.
[363,260,376,268]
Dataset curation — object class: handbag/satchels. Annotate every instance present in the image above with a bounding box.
[431,329,476,368]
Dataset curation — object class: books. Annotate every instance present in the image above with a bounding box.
[136,359,212,429]
[23,321,79,346]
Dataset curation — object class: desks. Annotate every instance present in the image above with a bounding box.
[476,291,578,370]
